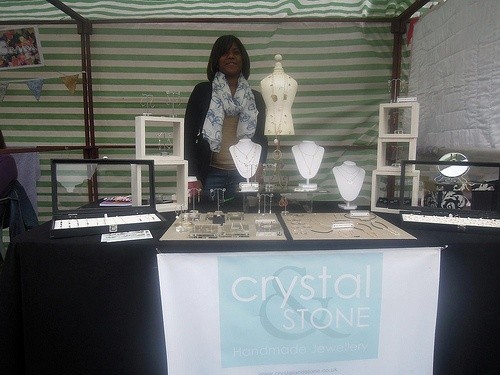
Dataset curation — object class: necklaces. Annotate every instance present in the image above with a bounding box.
[59,67,500,239]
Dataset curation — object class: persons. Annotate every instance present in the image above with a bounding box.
[184,35,268,214]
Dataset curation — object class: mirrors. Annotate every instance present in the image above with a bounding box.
[434,152,471,192]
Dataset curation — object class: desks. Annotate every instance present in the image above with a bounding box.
[0,197,500,375]
